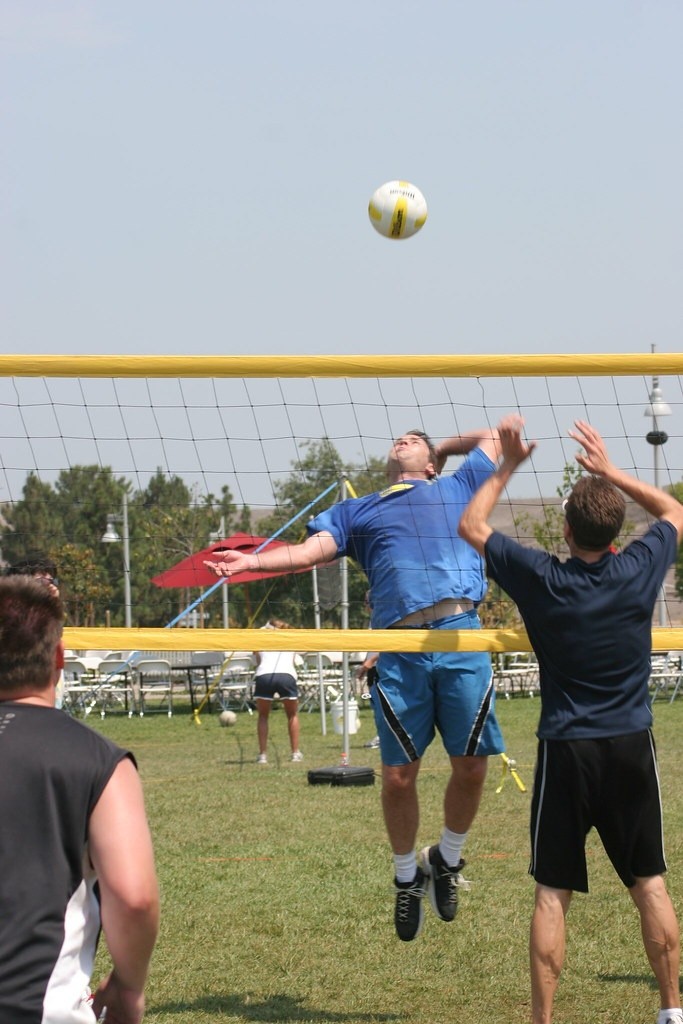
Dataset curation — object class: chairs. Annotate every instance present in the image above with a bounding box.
[495,652,540,700]
[62,650,368,719]
[649,652,683,705]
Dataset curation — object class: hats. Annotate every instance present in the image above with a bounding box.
[260,621,276,629]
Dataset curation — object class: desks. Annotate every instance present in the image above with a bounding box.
[116,664,211,712]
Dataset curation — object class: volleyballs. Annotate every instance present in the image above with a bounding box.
[367,180,428,240]
[220,710,237,726]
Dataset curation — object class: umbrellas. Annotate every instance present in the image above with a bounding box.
[151,533,337,629]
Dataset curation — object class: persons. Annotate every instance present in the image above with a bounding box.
[251,621,304,763]
[358,590,380,749]
[201,431,502,941]
[456,414,683,1024]
[1,575,159,1024]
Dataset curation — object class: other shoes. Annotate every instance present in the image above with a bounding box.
[291,752,301,762]
[257,754,266,763]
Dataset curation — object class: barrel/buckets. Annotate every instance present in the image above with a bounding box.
[330,700,359,735]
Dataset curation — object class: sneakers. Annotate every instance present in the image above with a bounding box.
[394,866,427,941]
[421,845,465,921]
[364,736,379,748]
